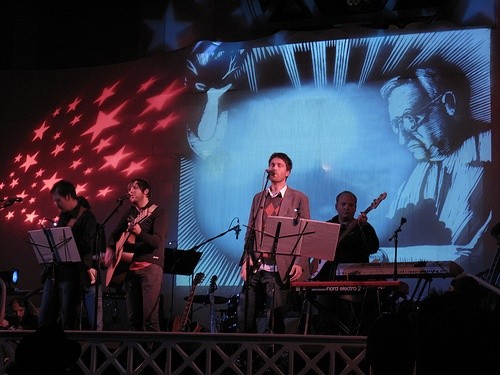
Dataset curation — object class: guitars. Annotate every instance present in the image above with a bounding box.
[172,272,206,333]
[308,193,387,279]
[106,203,159,289]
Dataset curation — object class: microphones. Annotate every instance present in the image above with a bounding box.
[118,193,132,202]
[236,219,239,239]
[265,170,275,176]
[8,198,23,202]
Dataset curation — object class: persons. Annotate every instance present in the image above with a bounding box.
[103,178,168,331]
[0,278,29,375]
[311,191,380,282]
[36,180,99,331]
[240,153,310,334]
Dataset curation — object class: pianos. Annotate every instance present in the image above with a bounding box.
[336,262,464,311]
[289,281,409,335]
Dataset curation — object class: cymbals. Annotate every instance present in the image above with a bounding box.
[215,308,236,313]
[183,295,229,304]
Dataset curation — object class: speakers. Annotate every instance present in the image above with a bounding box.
[5,289,136,330]
